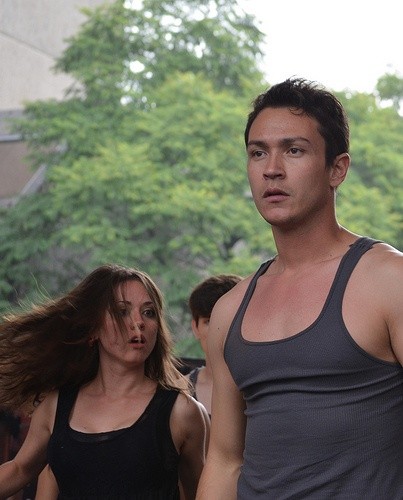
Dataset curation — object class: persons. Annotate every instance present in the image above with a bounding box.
[1,263,210,500]
[171,272,244,417]
[190,77,402,500]
[32,401,211,500]
